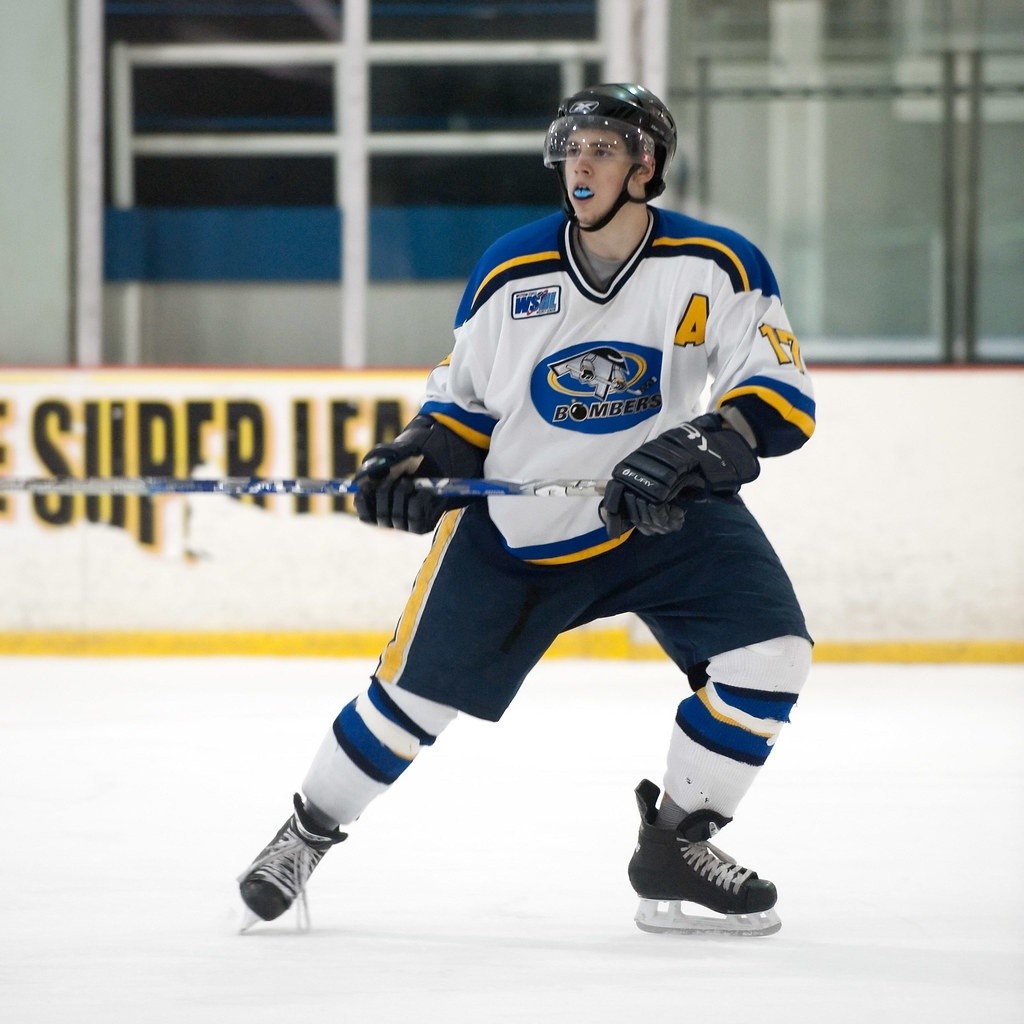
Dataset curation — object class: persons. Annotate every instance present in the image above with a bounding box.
[230,83,816,942]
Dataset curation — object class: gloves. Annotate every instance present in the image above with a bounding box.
[354,412,483,533]
[596,413,760,537]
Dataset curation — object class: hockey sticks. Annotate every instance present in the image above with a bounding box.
[0,475,612,503]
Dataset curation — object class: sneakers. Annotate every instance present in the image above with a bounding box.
[238,793,347,932]
[628,778,782,937]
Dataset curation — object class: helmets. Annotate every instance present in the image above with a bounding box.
[543,80,677,203]
